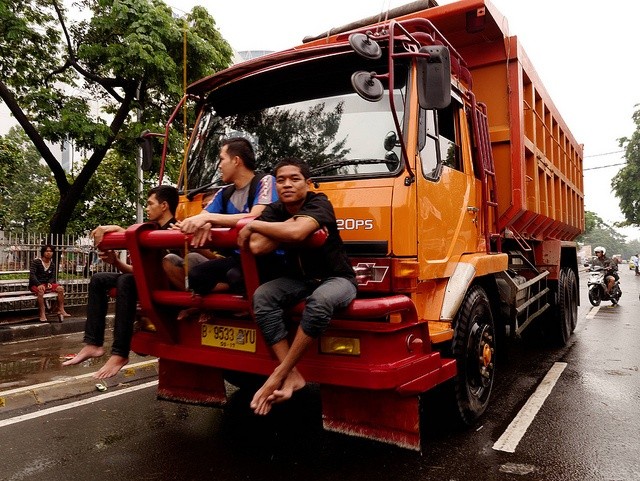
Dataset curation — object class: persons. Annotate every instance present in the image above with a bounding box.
[585,246,616,296]
[29,245,71,322]
[161,191,226,323]
[178,137,279,298]
[62,185,180,382]
[238,157,357,416]
[633,253,639,276]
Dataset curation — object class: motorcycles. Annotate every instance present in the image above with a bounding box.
[583,263,624,308]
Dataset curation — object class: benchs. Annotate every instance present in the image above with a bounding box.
[0,289,66,321]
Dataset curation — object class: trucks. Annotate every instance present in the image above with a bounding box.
[96,0,588,455]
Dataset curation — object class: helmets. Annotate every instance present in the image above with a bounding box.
[594,245,607,257]
